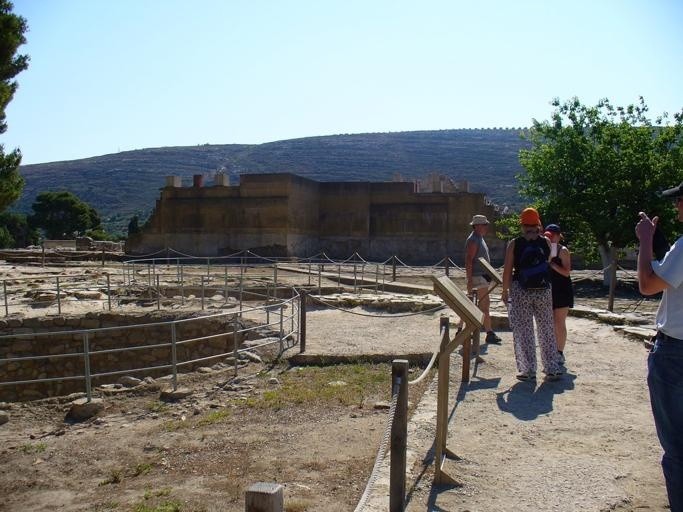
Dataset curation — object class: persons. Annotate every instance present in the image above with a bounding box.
[502,209,563,381]
[635,179,683,512]
[465,214,501,341]
[543,224,575,354]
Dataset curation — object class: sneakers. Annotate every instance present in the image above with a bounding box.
[516,354,565,380]
[486,335,501,342]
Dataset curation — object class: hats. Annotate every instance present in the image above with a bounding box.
[544,224,561,234]
[662,182,683,197]
[519,208,541,225]
[469,215,490,226]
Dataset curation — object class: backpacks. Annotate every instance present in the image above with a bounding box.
[519,245,550,290]
